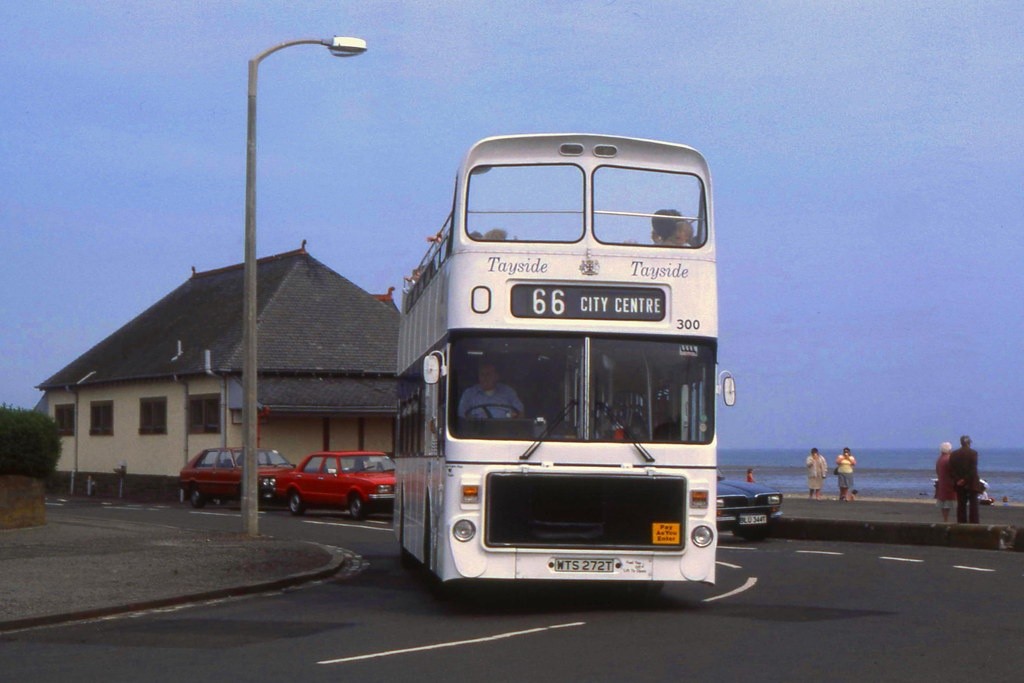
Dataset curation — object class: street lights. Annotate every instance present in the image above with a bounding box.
[241,33,370,537]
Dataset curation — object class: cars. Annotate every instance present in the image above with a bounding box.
[275,449,396,521]
[716,466,785,543]
[178,446,298,510]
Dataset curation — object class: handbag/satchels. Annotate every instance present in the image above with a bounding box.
[834,467,838,475]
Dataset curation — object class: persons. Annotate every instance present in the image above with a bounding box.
[934,434,983,523]
[834,447,856,503]
[746,468,755,483]
[650,208,695,247]
[403,228,508,283]
[805,448,828,502]
[457,362,524,421]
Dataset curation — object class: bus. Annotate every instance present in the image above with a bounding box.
[393,131,738,613]
